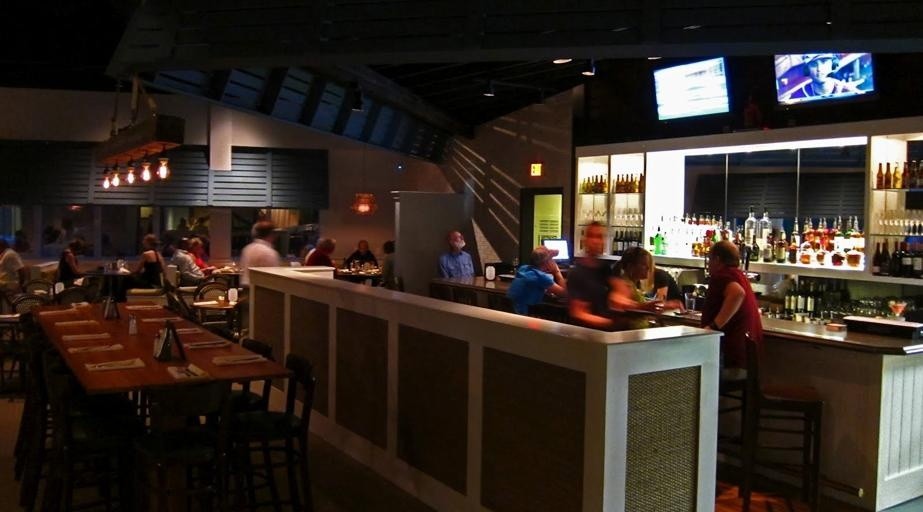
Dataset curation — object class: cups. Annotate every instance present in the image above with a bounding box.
[684,294,696,313]
[614,214,643,227]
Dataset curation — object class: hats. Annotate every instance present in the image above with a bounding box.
[531,247,558,264]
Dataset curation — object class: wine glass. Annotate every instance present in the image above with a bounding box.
[872,240,922,278]
[878,209,923,236]
[888,300,907,319]
[649,294,665,324]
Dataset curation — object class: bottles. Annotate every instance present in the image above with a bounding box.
[129,315,137,336]
[582,174,645,194]
[876,160,923,189]
[613,230,643,256]
[655,205,865,268]
[768,279,842,320]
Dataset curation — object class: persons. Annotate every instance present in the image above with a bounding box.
[506,246,567,316]
[239,219,395,344]
[436,230,475,305]
[0,229,33,305]
[169,217,209,248]
[790,56,860,102]
[58,241,85,283]
[636,265,686,310]
[561,220,665,332]
[700,240,764,370]
[130,234,217,288]
[612,246,687,330]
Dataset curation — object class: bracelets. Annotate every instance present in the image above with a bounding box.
[708,320,719,331]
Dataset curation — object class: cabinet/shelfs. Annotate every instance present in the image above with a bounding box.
[574,116,923,286]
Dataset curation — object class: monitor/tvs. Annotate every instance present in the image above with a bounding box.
[542,237,572,263]
[649,56,733,125]
[768,47,881,106]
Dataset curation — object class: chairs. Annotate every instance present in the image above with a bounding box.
[0,261,315,512]
[452,288,477,306]
[429,285,450,300]
[488,293,514,312]
[738,330,825,512]
[717,380,746,496]
[526,303,570,323]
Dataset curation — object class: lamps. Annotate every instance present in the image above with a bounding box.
[95,71,184,189]
[356,193,374,212]
[581,58,596,76]
[349,80,363,113]
[481,79,497,97]
[552,52,573,65]
